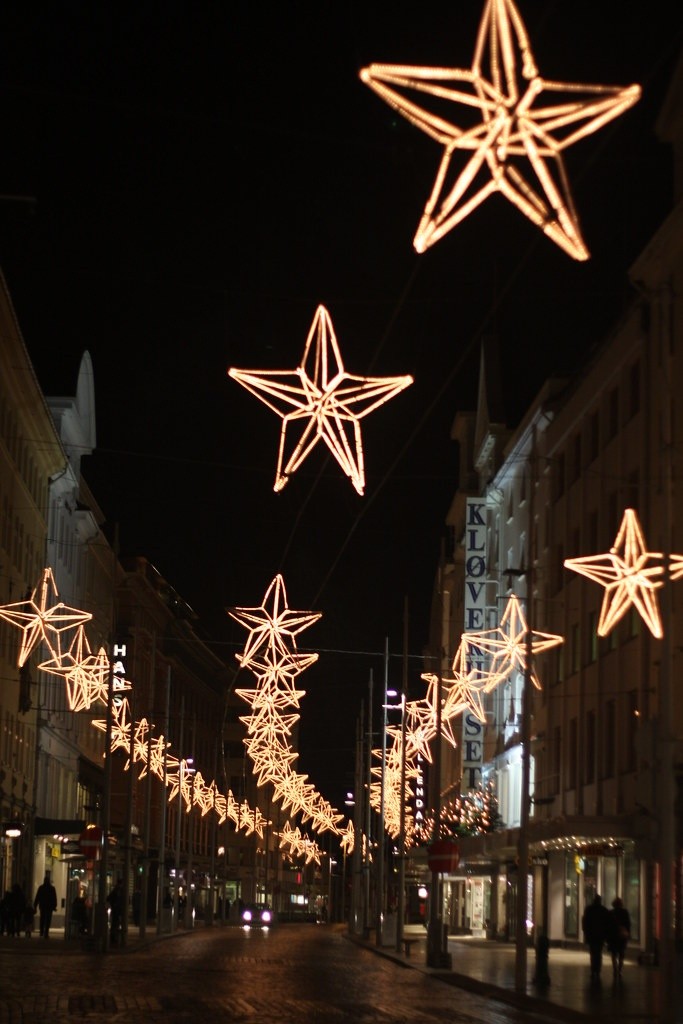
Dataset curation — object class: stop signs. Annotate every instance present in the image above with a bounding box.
[79,827,103,858]
[426,840,460,874]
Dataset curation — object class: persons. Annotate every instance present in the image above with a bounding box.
[164,891,187,920]
[107,878,123,945]
[133,893,141,926]
[0,876,57,937]
[581,894,632,979]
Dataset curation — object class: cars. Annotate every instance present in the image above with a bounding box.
[241,903,273,926]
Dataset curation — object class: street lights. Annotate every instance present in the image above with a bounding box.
[94,630,135,955]
[499,566,534,990]
[419,643,444,966]
[385,688,407,955]
